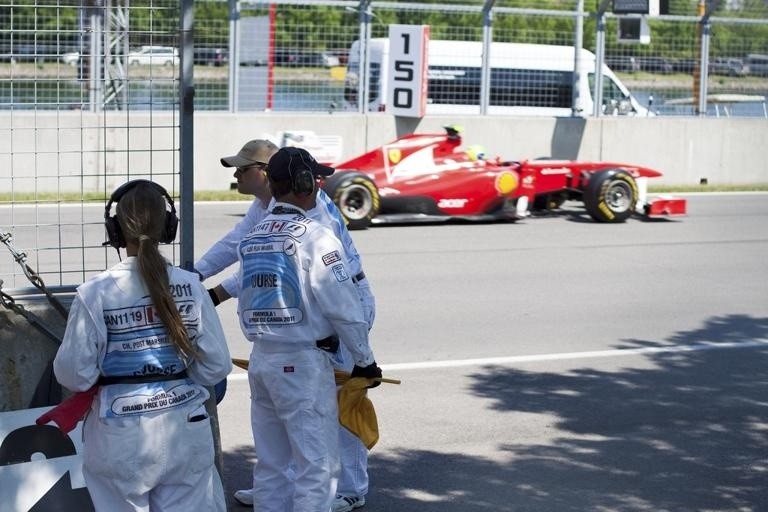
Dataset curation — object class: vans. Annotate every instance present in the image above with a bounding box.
[340,36,655,116]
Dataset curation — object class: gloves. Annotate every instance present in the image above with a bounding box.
[351,360,382,388]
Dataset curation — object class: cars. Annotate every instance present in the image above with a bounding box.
[605,52,767,78]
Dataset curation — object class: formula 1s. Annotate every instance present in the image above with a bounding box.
[308,124,689,231]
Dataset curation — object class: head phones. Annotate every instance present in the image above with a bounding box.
[281,146,316,199]
[102,178,179,250]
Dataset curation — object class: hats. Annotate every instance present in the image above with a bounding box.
[220,140,335,181]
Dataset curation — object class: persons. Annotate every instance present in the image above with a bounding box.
[465,147,487,162]
[441,122,464,136]
[193,139,375,512]
[237,147,383,512]
[53,179,232,512]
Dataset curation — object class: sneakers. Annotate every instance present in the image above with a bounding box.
[334,495,365,512]
[233,489,253,505]
